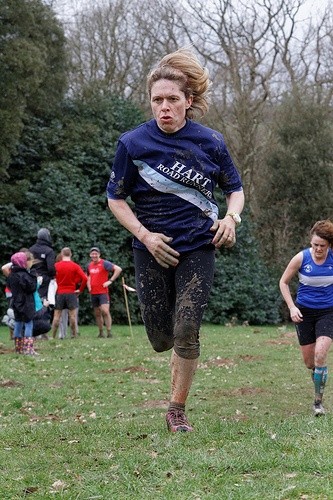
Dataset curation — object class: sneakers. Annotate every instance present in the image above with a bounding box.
[97,330,105,338]
[312,400,325,417]
[166,410,192,433]
[107,329,112,338]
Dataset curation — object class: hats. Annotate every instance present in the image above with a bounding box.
[38,228,52,243]
[90,247,100,252]
[11,252,28,269]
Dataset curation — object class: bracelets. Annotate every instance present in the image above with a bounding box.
[109,278,114,283]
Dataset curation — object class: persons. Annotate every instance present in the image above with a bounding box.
[7,251,39,356]
[37,272,58,339]
[27,227,60,341]
[50,247,88,340]
[87,247,122,338]
[0,247,43,338]
[54,251,79,339]
[105,51,245,432]
[278,220,333,419]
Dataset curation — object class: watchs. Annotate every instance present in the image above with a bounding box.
[225,210,243,226]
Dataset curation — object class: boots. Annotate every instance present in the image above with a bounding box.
[22,337,40,356]
[15,337,24,354]
